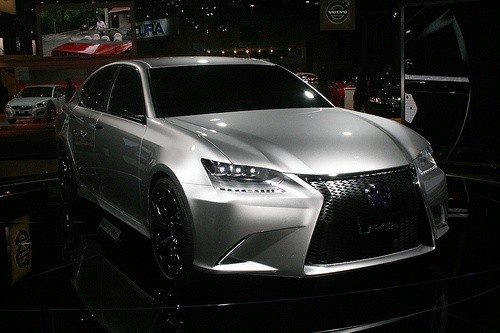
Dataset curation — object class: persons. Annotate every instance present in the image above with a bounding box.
[65,78,76,103]
[97,17,106,34]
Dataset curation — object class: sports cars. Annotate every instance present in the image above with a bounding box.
[50,28,133,57]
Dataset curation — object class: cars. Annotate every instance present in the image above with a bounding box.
[60,57,452,281]
[5,85,67,123]
